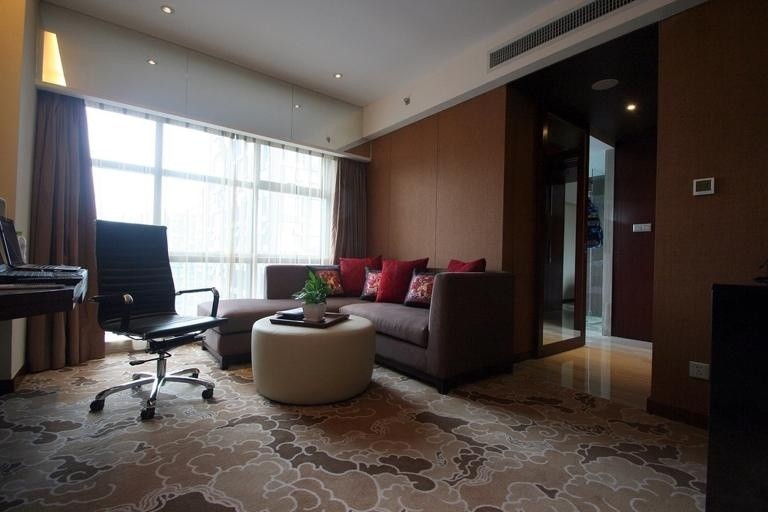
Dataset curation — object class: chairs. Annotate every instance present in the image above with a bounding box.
[87,220,230,421]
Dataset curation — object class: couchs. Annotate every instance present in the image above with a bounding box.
[196,265,514,395]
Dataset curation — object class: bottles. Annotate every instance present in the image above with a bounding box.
[15,230,26,264]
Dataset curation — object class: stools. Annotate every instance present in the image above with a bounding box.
[251,314,376,406]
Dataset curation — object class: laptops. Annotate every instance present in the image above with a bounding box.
[0,215,81,271]
[0,251,83,283]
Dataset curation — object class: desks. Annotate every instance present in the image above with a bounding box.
[0,265,87,322]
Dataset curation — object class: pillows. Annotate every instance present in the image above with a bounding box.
[447,258,486,272]
[404,268,447,307]
[359,265,382,300]
[310,266,342,294]
[338,254,383,297]
[375,257,429,303]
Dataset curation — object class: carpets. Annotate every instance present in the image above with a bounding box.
[1,344,707,512]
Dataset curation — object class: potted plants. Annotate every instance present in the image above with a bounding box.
[292,266,333,321]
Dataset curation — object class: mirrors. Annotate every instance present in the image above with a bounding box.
[534,111,585,359]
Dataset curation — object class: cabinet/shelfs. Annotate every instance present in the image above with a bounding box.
[706,280,767,512]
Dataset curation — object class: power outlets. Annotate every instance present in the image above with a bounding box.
[689,361,711,381]
